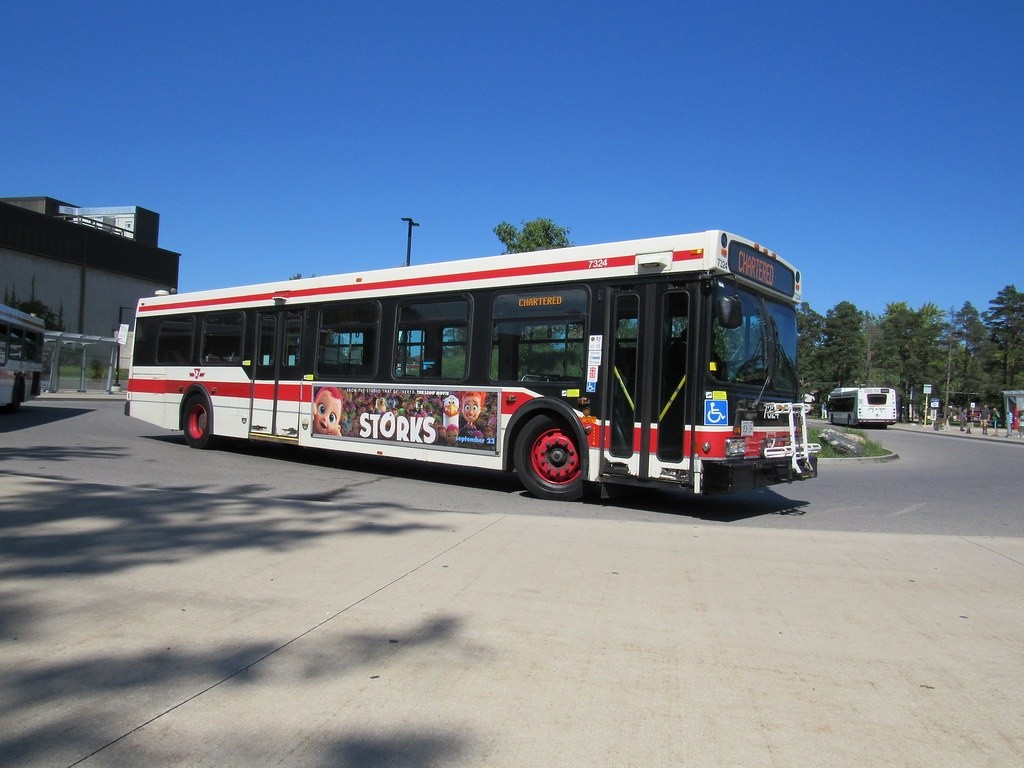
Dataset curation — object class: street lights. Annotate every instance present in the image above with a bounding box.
[401,216,421,378]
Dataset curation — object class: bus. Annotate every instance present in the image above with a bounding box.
[0,300,47,412]
[826,382,897,429]
[121,231,819,498]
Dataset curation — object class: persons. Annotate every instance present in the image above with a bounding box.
[958,409,968,430]
[978,404,990,434]
[1018,412,1024,436]
[821,401,827,419]
[1005,411,1013,433]
[991,408,1000,435]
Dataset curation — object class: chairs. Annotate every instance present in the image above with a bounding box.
[206,351,241,365]
